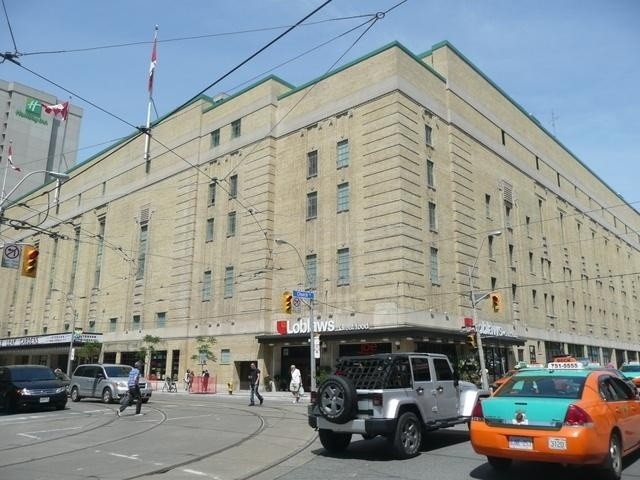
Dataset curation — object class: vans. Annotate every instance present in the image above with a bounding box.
[69,363,152,403]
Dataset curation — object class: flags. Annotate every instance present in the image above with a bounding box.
[147,32,158,90]
[41,102,68,121]
[8,146,21,172]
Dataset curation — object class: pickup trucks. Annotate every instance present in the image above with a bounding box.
[308,350,490,457]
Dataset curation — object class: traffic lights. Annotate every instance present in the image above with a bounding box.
[281,291,292,315]
[21,245,39,277]
[490,292,500,312]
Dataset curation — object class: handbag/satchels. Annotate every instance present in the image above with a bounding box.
[298,386,304,396]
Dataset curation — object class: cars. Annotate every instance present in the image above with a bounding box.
[469,357,640,480]
[1,364,68,412]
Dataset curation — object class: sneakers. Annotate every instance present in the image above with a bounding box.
[116,408,144,418]
[248,399,263,406]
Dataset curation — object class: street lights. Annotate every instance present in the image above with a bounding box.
[50,289,75,379]
[0,170,69,205]
[469,231,502,390]
[275,238,319,393]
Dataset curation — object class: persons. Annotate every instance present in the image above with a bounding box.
[117,361,144,417]
[290,365,303,404]
[248,363,264,405]
[161,369,209,392]
